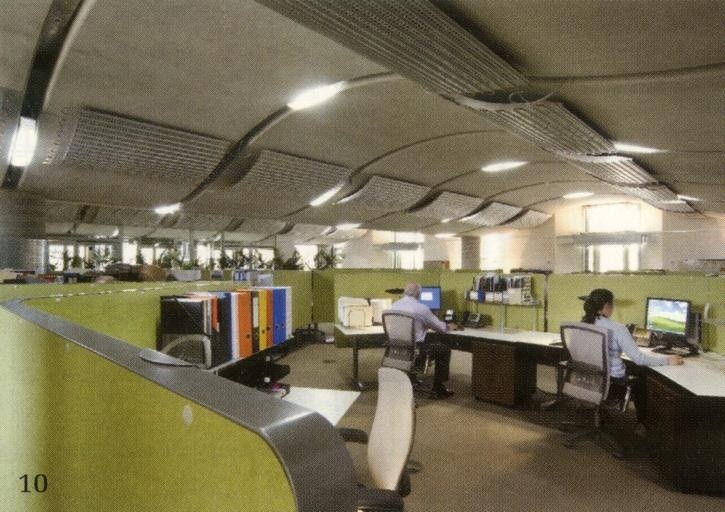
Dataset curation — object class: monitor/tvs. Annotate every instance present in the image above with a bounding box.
[418,287,442,311]
[644,297,692,355]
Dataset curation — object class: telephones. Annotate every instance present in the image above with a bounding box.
[625,324,653,347]
[460,311,483,329]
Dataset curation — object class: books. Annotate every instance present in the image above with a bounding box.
[473,272,524,293]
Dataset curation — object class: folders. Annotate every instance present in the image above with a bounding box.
[161,286,293,370]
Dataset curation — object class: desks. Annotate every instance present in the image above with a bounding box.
[334,325,725,492]
[253,385,361,427]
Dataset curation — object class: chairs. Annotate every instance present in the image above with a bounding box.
[381,311,434,394]
[335,365,415,512]
[557,323,642,461]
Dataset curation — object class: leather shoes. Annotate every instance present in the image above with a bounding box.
[410,379,422,384]
[432,385,453,395]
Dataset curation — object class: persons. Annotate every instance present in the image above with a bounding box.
[391,282,455,396]
[580,289,685,438]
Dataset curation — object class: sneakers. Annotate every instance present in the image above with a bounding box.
[634,423,647,438]
